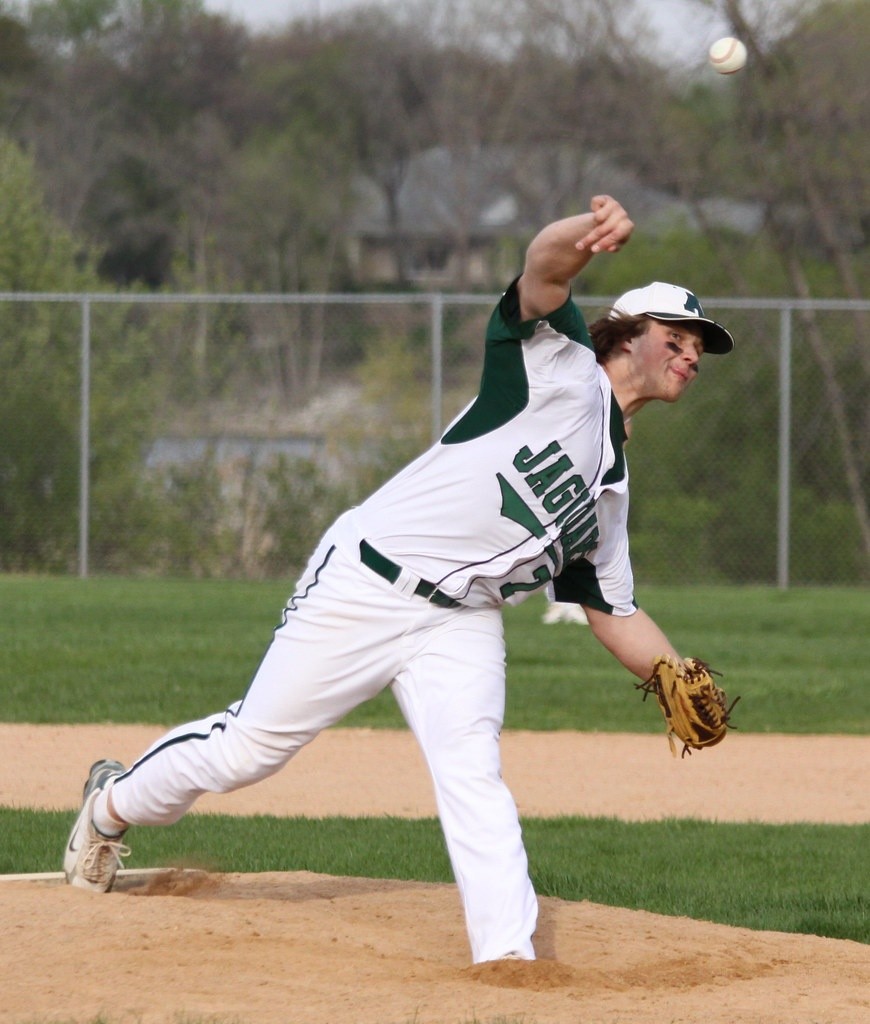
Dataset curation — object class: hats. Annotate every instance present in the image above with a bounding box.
[608,282,735,355]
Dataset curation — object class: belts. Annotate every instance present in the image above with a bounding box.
[360,539,461,608]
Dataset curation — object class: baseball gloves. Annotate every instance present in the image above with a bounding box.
[632,653,741,759]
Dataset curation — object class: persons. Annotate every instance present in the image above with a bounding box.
[63,194,735,962]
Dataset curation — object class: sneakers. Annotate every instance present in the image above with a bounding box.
[62,760,127,894]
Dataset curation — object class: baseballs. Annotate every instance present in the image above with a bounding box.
[709,37,748,76]
